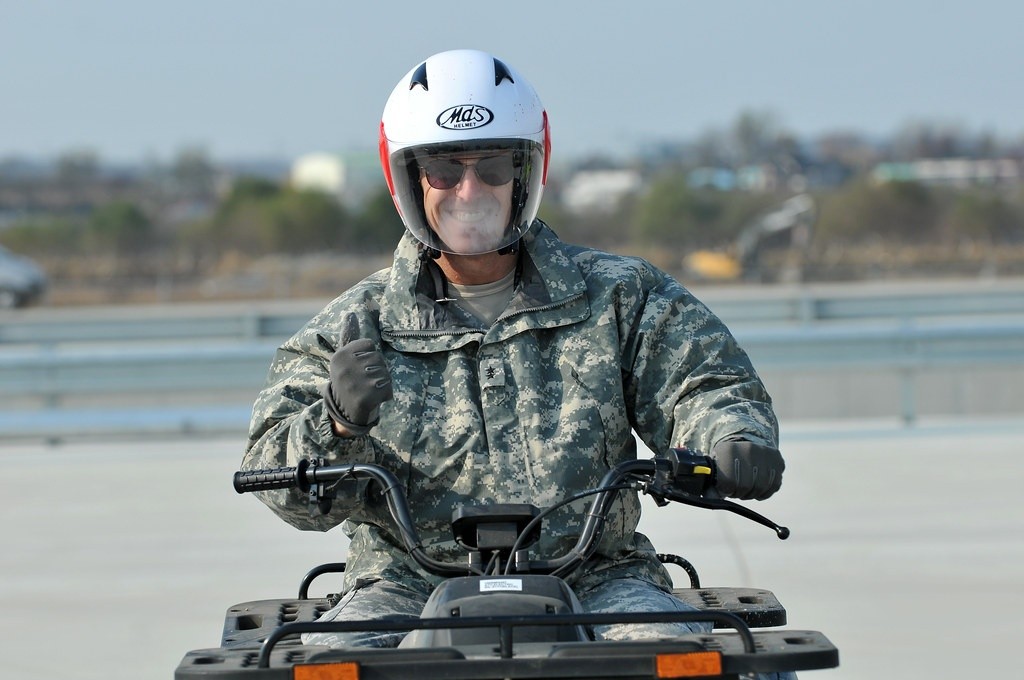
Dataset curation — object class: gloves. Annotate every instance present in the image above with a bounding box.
[323,312,394,436]
[703,436,785,500]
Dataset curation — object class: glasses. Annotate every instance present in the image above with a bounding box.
[418,155,515,189]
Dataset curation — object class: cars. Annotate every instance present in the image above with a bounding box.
[0,247,50,313]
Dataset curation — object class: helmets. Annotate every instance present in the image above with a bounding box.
[378,50,551,255]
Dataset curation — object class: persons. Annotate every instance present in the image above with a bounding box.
[240,51,790,657]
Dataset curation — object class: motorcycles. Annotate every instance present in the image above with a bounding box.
[175,447,842,680]
